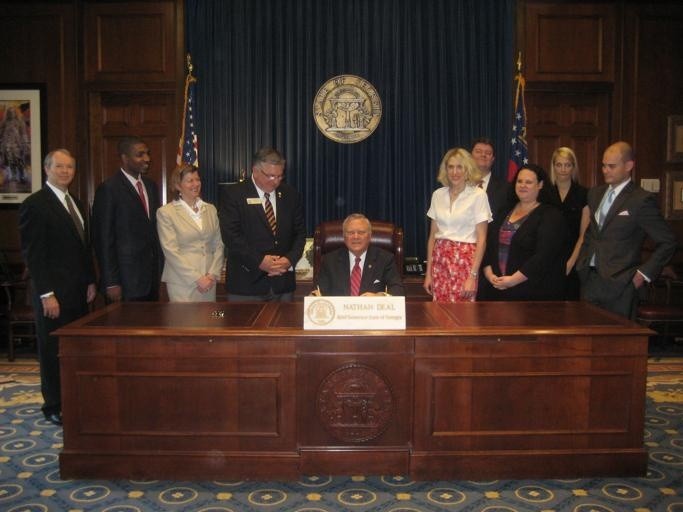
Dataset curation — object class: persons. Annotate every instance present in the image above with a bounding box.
[572,142,674,323]
[154,162,224,302]
[482,164,565,300]
[466,140,511,299]
[309,215,404,296]
[93,140,161,302]
[538,147,590,299]
[423,148,493,303]
[218,148,306,300]
[16,150,97,424]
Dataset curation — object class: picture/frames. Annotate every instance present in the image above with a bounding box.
[1,83,47,210]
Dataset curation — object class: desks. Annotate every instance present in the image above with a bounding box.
[55,301,658,484]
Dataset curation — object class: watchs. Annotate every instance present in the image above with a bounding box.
[470,272,478,277]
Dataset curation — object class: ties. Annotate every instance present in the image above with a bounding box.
[477,177,484,189]
[599,188,615,229]
[65,193,86,240]
[264,192,279,237]
[137,182,150,218]
[351,256,362,296]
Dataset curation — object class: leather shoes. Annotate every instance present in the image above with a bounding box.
[44,409,62,425]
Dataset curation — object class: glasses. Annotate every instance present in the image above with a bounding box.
[258,166,286,181]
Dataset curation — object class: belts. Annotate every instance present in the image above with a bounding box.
[590,266,596,271]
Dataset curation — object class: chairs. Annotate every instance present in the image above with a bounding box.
[635,265,683,354]
[4,268,40,361]
[313,223,404,299]
